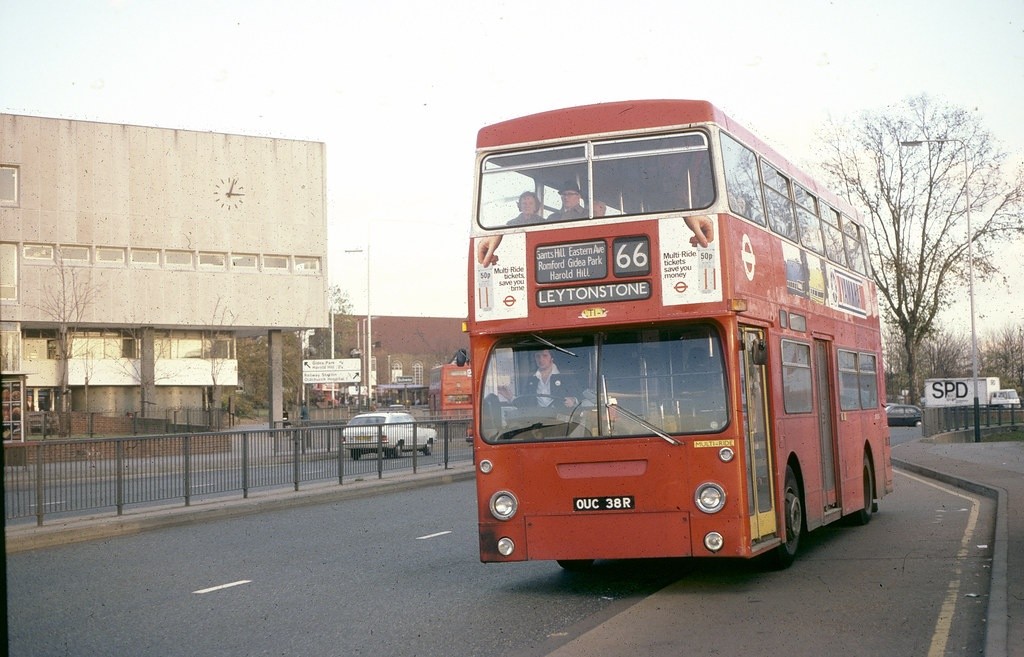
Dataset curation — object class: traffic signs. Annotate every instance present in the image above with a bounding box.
[302,358,362,383]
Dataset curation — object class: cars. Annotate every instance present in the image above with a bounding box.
[343,412,438,460]
[887,405,922,427]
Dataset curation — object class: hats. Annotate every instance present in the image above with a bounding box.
[558,181,579,194]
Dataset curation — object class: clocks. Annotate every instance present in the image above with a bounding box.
[213,175,246,211]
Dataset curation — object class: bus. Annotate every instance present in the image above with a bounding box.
[428,363,473,421]
[467,98,893,570]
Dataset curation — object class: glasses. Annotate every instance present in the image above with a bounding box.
[561,193,577,196]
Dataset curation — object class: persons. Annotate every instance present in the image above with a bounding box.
[27,395,34,411]
[593,201,607,219]
[299,402,310,433]
[548,181,588,223]
[496,347,583,411]
[503,190,547,222]
[282,408,289,428]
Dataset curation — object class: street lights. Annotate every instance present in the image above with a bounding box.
[345,245,371,409]
[902,139,981,442]
[362,316,380,407]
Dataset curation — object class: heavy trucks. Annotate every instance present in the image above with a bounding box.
[925,377,1021,409]
